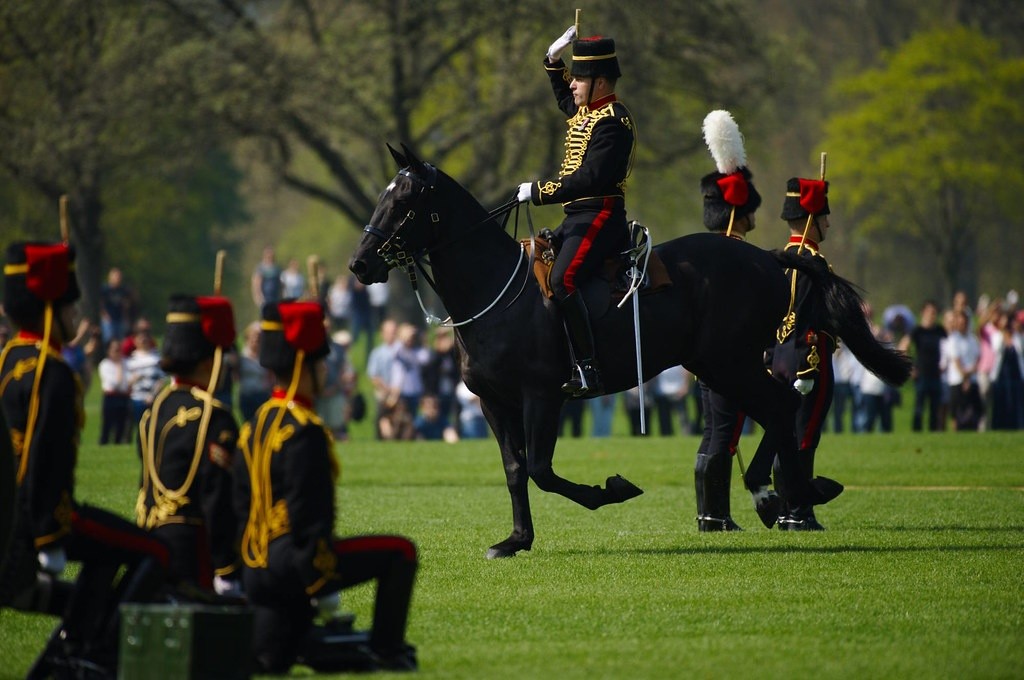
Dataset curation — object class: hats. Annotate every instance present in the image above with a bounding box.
[258,255,329,372]
[571,9,621,79]
[3,194,80,306]
[165,251,235,348]
[781,152,830,221]
[701,109,760,230]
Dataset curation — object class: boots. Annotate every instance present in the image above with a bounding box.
[559,291,604,397]
[773,449,824,529]
[693,456,739,532]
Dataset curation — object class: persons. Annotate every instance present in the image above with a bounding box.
[694,111,766,532]
[822,287,1024,432]
[1,240,420,679]
[514,25,636,394]
[555,362,702,436]
[366,317,490,444]
[775,179,837,528]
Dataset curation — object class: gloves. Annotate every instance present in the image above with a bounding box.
[214,572,245,600]
[546,27,576,62]
[39,545,66,575]
[517,182,534,202]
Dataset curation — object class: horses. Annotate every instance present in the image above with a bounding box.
[349,143,915,561]
